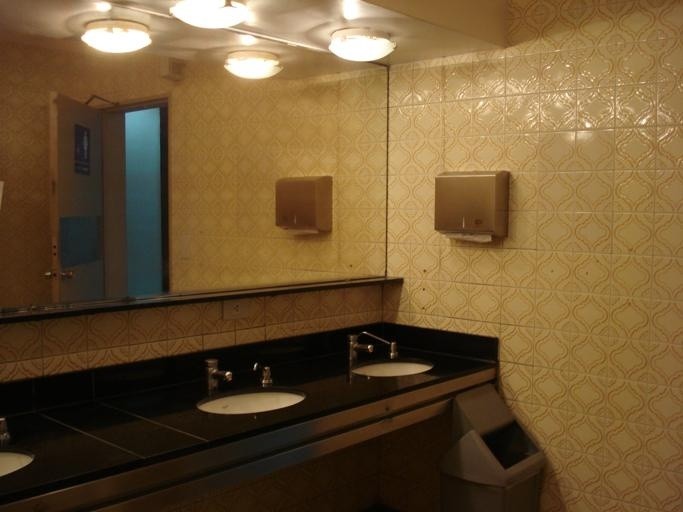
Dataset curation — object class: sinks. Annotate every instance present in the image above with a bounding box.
[354,359,436,379]
[0,447,35,478]
[193,386,308,418]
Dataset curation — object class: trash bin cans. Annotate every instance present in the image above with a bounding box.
[434,382,547,512]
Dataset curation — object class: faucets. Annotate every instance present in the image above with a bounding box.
[346,333,374,386]
[203,358,235,396]
[0,417,11,450]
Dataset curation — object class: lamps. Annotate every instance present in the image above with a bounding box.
[169,1,254,32]
[327,27,396,64]
[221,51,284,80]
[79,19,152,54]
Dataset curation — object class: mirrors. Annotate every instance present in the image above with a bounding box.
[0,1,390,324]
